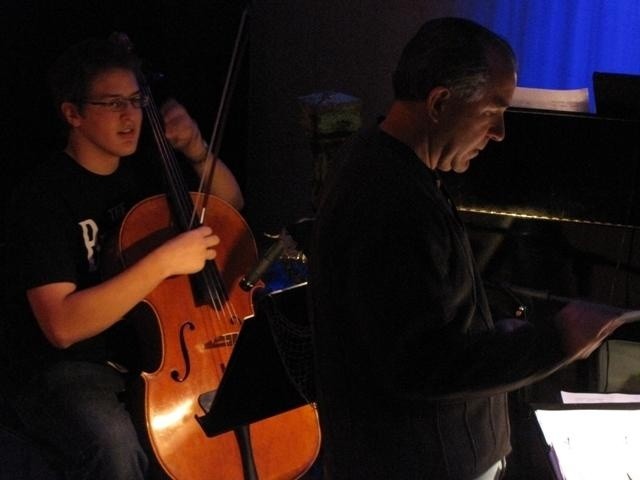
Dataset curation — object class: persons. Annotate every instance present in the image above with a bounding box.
[12,26,247,479]
[308,15,631,477]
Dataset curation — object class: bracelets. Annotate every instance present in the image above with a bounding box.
[188,143,209,163]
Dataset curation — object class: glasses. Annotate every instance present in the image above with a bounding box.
[84,94,151,112]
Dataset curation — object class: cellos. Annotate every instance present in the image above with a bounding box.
[105,4,323,480]
